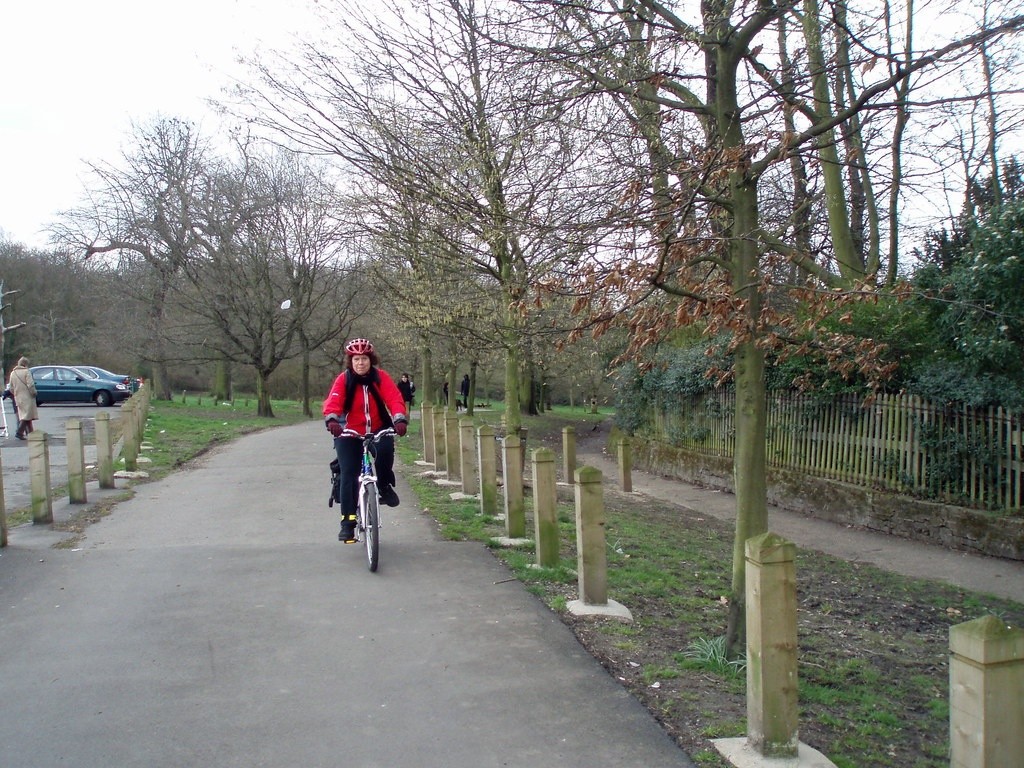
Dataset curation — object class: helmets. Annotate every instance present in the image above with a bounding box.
[346,338,374,355]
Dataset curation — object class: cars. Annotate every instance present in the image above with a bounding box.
[41,366,131,394]
[6,365,131,407]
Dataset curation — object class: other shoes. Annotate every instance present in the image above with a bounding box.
[337,520,358,541]
[376,481,400,507]
[15,432,26,440]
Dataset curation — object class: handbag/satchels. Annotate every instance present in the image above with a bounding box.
[328,457,341,506]
[36,393,45,406]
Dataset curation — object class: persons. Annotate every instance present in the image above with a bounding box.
[396,373,415,411]
[461,374,469,412]
[323,339,408,541]
[3,356,38,440]
[444,382,449,403]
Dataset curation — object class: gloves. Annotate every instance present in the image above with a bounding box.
[393,419,408,437]
[325,418,344,437]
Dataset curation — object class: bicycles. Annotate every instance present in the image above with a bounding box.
[326,426,398,573]
